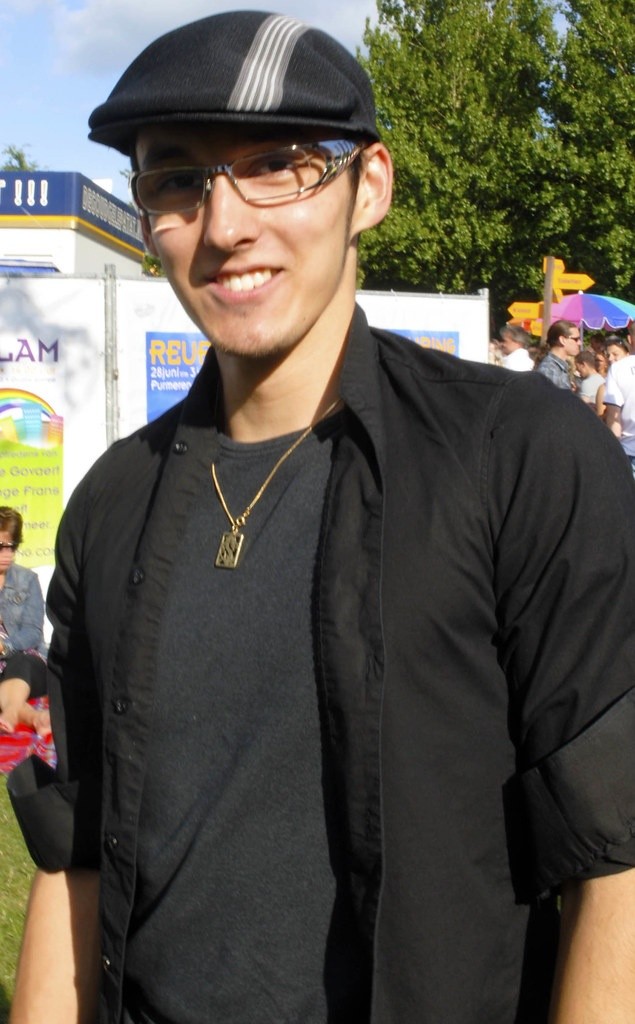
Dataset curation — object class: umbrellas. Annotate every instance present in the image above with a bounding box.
[538,292,635,352]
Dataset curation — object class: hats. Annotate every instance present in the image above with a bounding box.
[89,12,380,152]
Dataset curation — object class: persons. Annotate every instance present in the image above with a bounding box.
[0,11,635,1024]
[0,505,52,742]
[489,321,635,480]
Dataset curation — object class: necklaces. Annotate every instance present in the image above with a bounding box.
[208,395,343,568]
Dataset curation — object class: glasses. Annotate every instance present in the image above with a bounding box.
[564,335,580,342]
[0,542,17,550]
[607,340,629,352]
[130,139,371,215]
